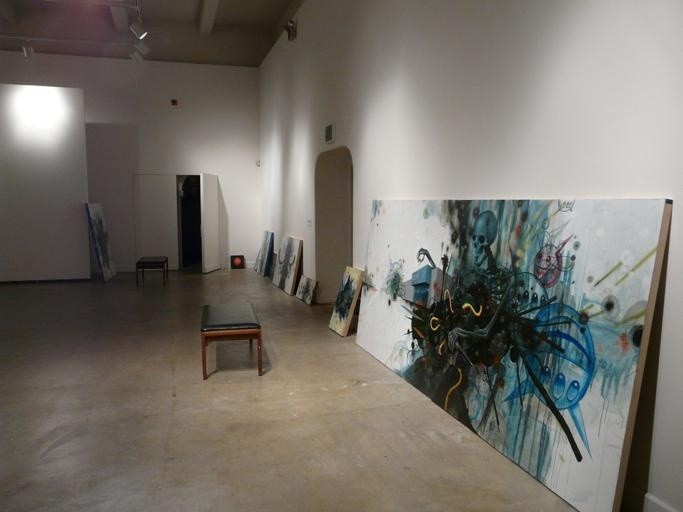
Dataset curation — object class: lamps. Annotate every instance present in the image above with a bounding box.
[22,40,34,58]
[128,7,148,40]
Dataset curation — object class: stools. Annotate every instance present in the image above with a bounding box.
[200,303,263,380]
[136,256,168,288]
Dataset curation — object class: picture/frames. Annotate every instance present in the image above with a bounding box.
[323,121,335,144]
[85,202,117,282]
[295,275,319,306]
[354,198,674,512]
[254,230,274,277]
[230,255,244,269]
[272,233,303,296]
[328,266,363,337]
